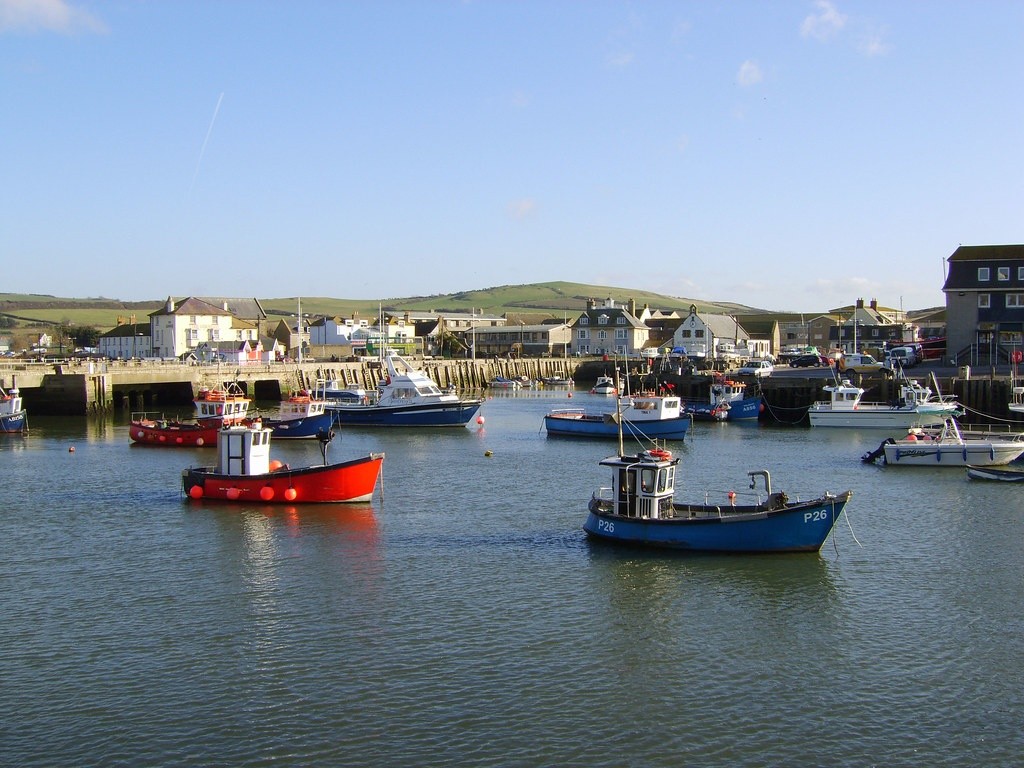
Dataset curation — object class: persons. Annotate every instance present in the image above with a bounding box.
[894,356,903,379]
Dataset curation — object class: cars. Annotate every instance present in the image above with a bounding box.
[820,356,834,367]
[737,360,774,378]
[789,353,822,369]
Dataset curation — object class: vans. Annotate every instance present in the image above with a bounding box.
[885,347,917,370]
[903,343,924,364]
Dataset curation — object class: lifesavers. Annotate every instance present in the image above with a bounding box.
[724,380,734,384]
[588,498,614,517]
[990,449,995,461]
[962,449,967,461]
[205,390,221,401]
[896,449,900,461]
[183,473,205,498]
[937,449,941,462]
[643,449,672,461]
[21,432,29,439]
[828,359,834,365]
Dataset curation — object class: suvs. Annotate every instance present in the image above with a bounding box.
[838,353,887,379]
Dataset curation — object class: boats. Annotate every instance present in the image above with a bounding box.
[293,304,487,428]
[0,374,30,435]
[127,339,253,448]
[540,351,694,442]
[966,463,1024,483]
[582,350,854,555]
[485,370,575,389]
[679,361,764,423]
[253,364,341,440]
[181,375,385,506]
[808,355,967,430]
[860,370,1024,466]
[591,368,615,394]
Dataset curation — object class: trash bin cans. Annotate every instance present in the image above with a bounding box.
[582,351,586,358]
[192,361,197,366]
[603,354,608,362]
[958,365,971,380]
[494,355,498,363]
[197,361,201,366]
